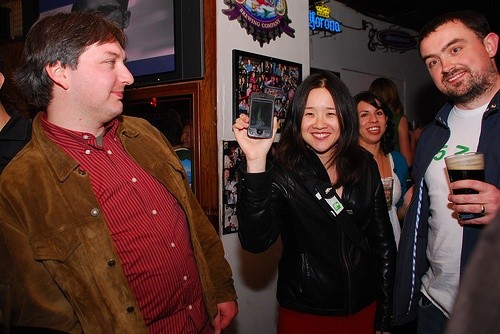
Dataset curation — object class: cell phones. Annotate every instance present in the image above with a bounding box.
[247,92,275,139]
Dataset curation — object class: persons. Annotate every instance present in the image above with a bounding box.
[370,75,410,159]
[395,10,499,334]
[350,91,412,246]
[222,55,300,231]
[233,72,398,334]
[0,57,33,175]
[0,13,238,334]
[70,1,131,48]
[176,122,193,149]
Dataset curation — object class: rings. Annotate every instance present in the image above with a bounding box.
[479,205,486,216]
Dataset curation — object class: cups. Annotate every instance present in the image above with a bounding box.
[380,177,393,211]
[444,153,487,219]
